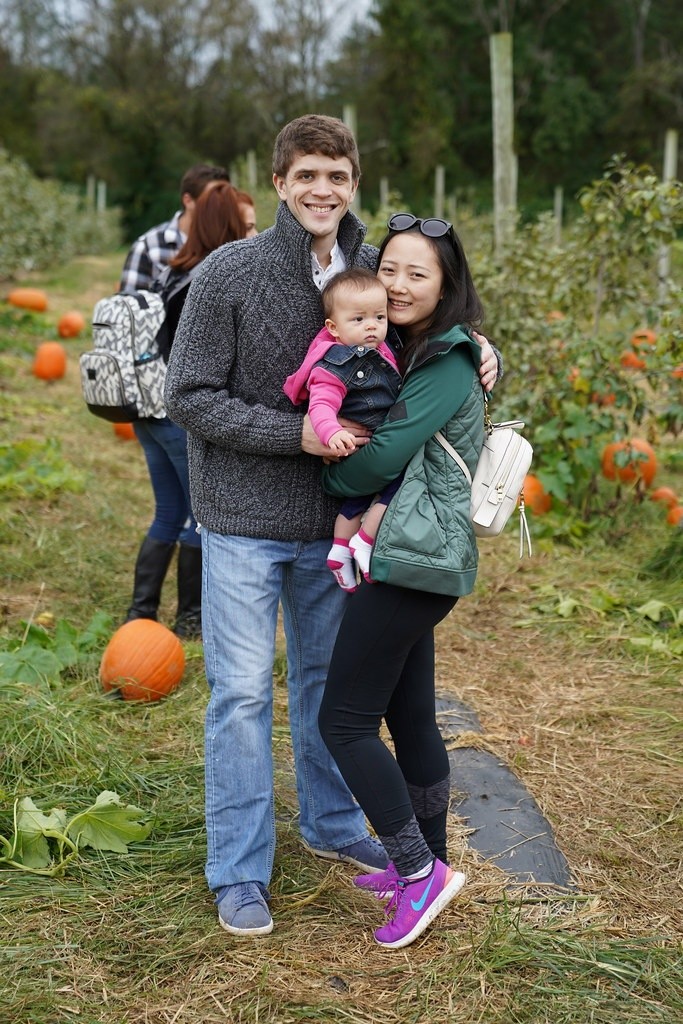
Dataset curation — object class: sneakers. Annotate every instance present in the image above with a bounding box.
[213,882,274,937]
[373,857,468,950]
[352,860,399,899]
[303,830,392,874]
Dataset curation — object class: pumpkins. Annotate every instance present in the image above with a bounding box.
[549,311,683,404]
[115,423,133,439]
[101,619,184,701]
[59,312,83,337]
[667,507,683,524]
[34,342,65,380]
[517,475,551,516]
[603,438,656,487]
[8,289,47,310]
[653,488,677,507]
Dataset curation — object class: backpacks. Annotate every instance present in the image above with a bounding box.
[432,391,534,538]
[77,261,205,424]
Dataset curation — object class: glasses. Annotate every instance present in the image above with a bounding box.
[386,212,455,238]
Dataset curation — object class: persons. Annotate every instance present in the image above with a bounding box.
[319,211,489,950]
[163,112,505,937]
[283,271,408,591]
[117,163,260,643]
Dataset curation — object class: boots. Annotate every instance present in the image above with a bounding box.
[173,541,201,642]
[122,536,178,626]
